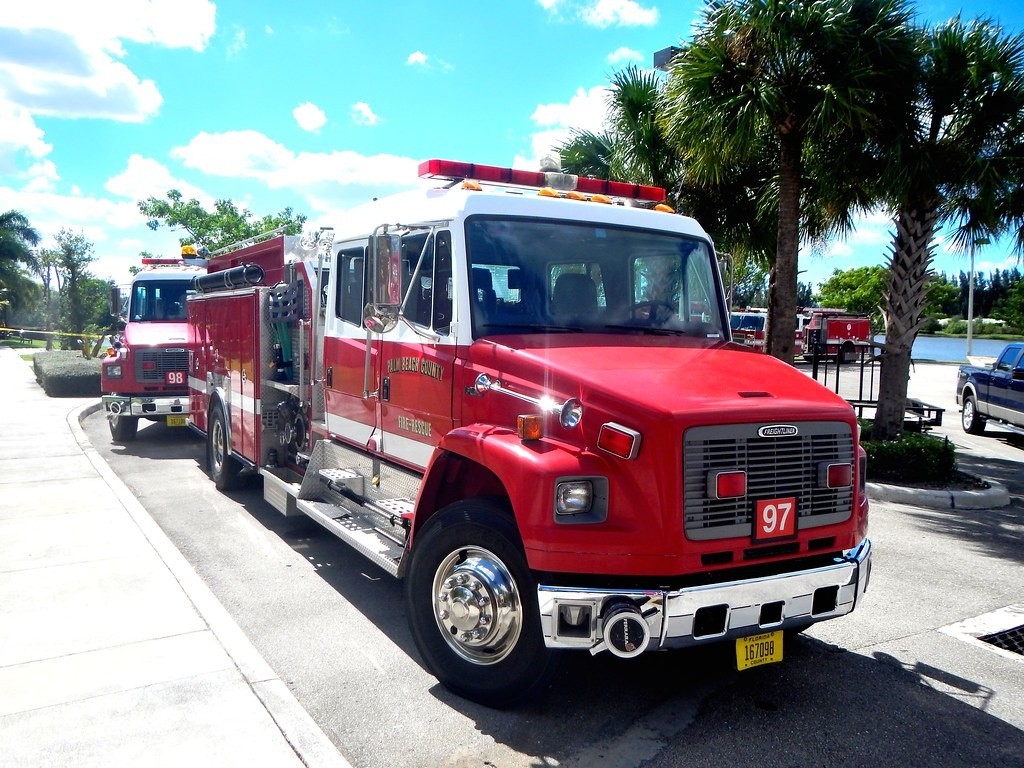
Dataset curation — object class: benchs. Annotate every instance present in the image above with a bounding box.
[845,397,945,425]
[20,338,32,345]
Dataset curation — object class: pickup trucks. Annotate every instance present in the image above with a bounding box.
[956,342,1024,437]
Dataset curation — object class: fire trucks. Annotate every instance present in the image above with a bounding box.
[99,243,212,442]
[687,299,872,364]
[177,158,872,701]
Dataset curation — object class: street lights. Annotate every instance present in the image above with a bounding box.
[964,237,991,356]
